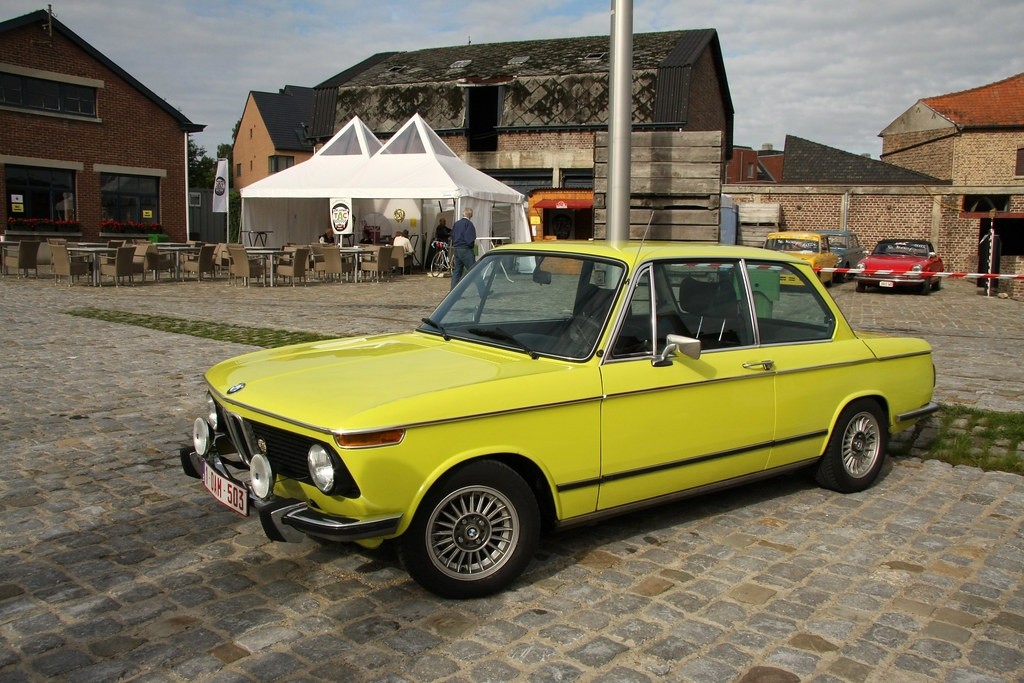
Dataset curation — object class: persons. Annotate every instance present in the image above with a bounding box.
[318,228,414,262]
[450,208,494,298]
[435,215,453,246]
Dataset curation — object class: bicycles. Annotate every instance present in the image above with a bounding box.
[430,240,466,281]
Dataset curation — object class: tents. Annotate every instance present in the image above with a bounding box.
[239,113,532,247]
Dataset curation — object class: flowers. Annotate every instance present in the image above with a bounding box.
[100,220,164,231]
[7,218,80,228]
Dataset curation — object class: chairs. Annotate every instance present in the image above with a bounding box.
[679,276,739,348]
[580,284,632,354]
[0,239,414,288]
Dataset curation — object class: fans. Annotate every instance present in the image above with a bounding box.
[355,213,393,282]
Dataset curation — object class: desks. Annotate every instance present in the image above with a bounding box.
[476,237,515,283]
[339,247,365,282]
[246,250,296,287]
[340,249,378,284]
[77,243,108,262]
[245,246,281,278]
[240,230,274,260]
[67,247,118,287]
[153,243,192,273]
[157,247,201,282]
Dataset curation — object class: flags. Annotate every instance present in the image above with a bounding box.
[212,160,228,212]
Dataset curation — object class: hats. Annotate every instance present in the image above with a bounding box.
[399,229,410,237]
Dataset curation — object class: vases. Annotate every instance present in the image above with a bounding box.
[11,225,79,232]
[101,228,163,234]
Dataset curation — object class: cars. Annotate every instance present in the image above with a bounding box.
[176,241,943,600]
[854,237,944,295]
[762,230,838,288]
[812,231,868,283]
